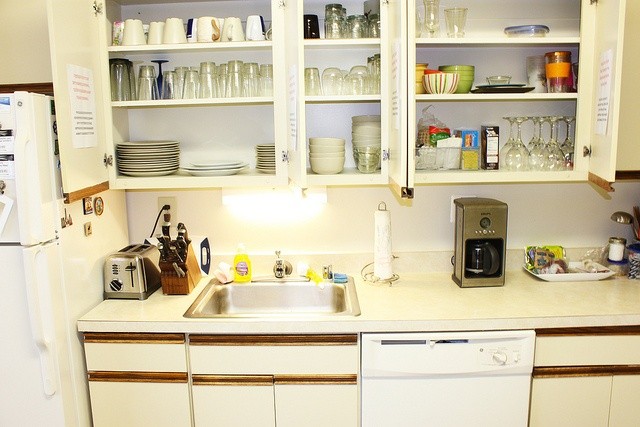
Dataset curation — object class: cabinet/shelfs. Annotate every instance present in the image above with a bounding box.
[598,0,640,192]
[187,333,361,426]
[528,325,640,426]
[83,332,193,426]
[288,1,407,199]
[46,0,288,203]
[407,1,598,199]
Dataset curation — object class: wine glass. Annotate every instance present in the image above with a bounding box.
[501,116,575,171]
[424,0,440,38]
[151,60,169,98]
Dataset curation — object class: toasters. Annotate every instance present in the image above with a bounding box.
[103,243,162,299]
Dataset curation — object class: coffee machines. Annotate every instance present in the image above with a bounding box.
[451,197,508,287]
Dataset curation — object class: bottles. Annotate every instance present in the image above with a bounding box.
[480,125,500,169]
[546,51,573,92]
[609,238,626,261]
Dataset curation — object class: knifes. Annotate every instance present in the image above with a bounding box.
[156,220,190,277]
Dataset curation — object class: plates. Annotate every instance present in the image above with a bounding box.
[259,146,277,172]
[116,140,179,176]
[529,262,614,281]
[181,161,250,175]
[472,84,536,94]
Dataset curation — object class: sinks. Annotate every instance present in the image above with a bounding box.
[193,284,348,316]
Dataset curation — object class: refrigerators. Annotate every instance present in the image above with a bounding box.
[1,90,131,427]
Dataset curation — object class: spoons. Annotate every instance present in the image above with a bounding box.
[611,210,635,234]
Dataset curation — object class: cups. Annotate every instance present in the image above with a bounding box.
[245,15,266,40]
[121,19,145,45]
[368,54,381,95]
[163,17,187,43]
[221,17,245,42]
[525,55,547,93]
[303,14,321,39]
[322,66,370,96]
[109,58,135,100]
[305,67,321,96]
[138,65,156,99]
[165,60,273,99]
[444,7,468,38]
[197,17,220,42]
[147,22,166,45]
[325,3,381,39]
[187,18,198,43]
[112,61,136,100]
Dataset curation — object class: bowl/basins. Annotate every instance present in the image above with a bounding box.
[486,75,512,84]
[351,114,381,173]
[438,65,474,94]
[416,63,428,94]
[421,69,459,94]
[309,137,346,174]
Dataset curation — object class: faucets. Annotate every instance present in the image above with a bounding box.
[273,251,292,279]
